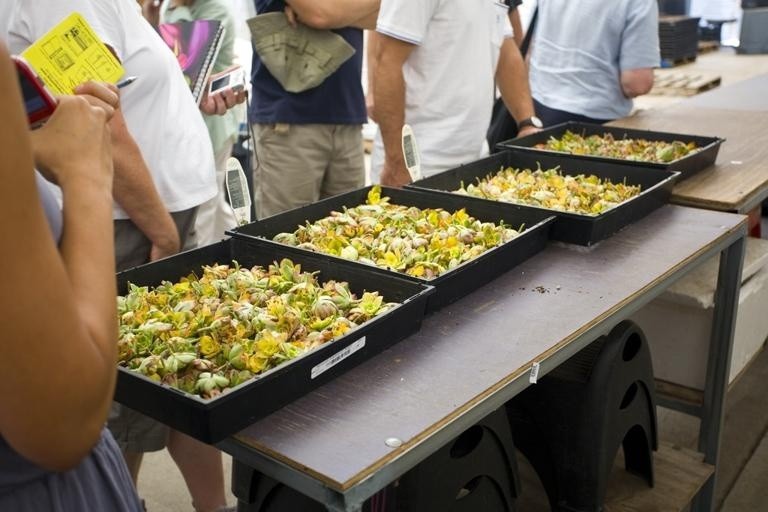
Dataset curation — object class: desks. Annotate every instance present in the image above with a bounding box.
[209,71,768,512]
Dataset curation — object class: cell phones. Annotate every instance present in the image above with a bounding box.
[11,55,59,130]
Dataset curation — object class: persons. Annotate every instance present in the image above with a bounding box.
[1,1,246,512]
[247,0,381,221]
[527,0,662,128]
[1,36,147,512]
[140,1,246,248]
[365,0,545,188]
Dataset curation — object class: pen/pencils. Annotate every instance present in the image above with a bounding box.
[117,78,137,88]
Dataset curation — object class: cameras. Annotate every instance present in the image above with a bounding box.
[208,66,245,97]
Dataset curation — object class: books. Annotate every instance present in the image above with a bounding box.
[158,18,225,107]
